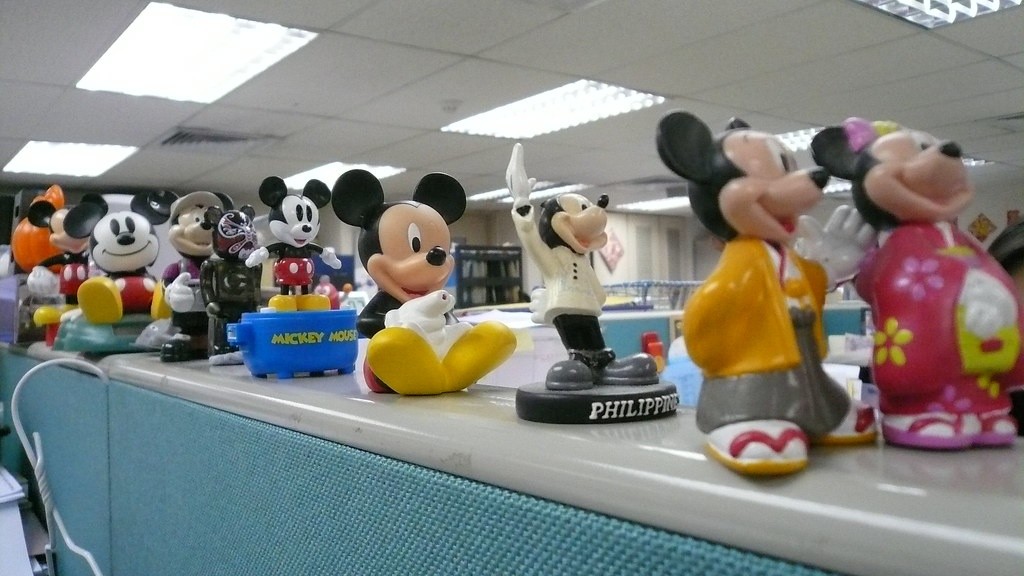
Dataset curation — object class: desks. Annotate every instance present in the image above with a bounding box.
[0,301,1024,576]
